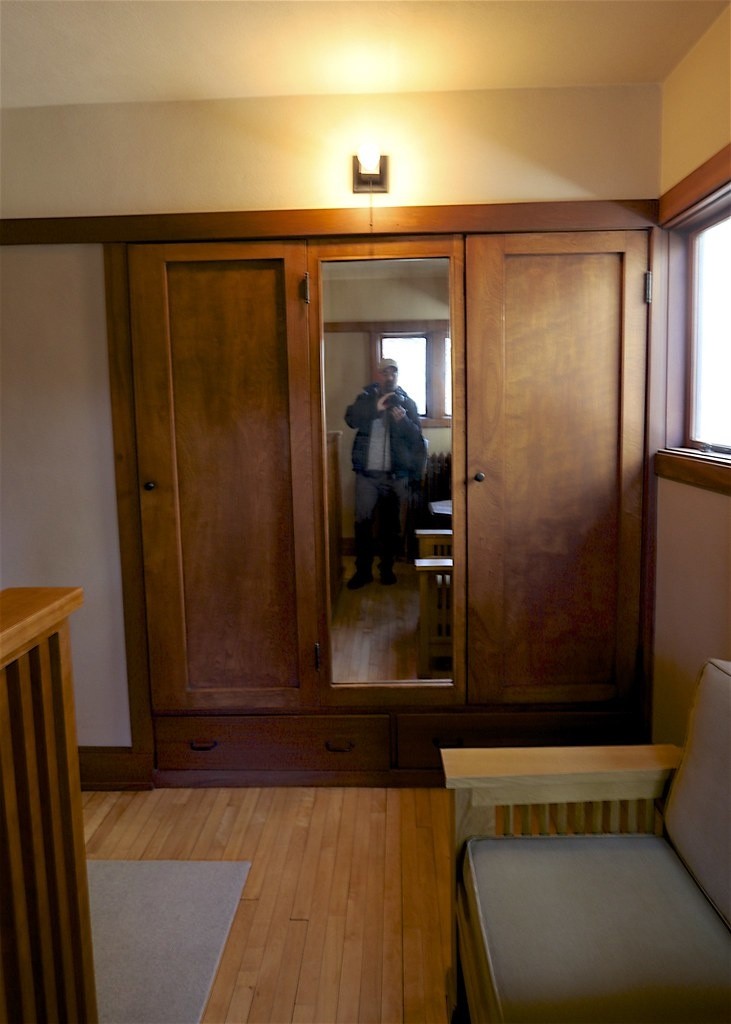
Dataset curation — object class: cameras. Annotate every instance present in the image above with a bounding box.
[391,394,407,408]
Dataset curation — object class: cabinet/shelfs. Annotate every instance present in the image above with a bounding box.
[102,229,653,790]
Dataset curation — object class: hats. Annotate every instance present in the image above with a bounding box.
[378,359,398,370]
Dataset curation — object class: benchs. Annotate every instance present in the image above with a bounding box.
[440,658,731,1024]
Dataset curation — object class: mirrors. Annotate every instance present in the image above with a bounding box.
[320,255,457,688]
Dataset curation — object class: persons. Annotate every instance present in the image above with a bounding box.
[344,358,425,590]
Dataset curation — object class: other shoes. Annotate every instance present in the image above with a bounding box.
[377,563,397,585]
[347,572,374,590]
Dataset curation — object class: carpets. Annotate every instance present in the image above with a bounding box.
[86,858,253,1024]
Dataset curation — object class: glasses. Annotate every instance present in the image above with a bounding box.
[379,371,398,378]
